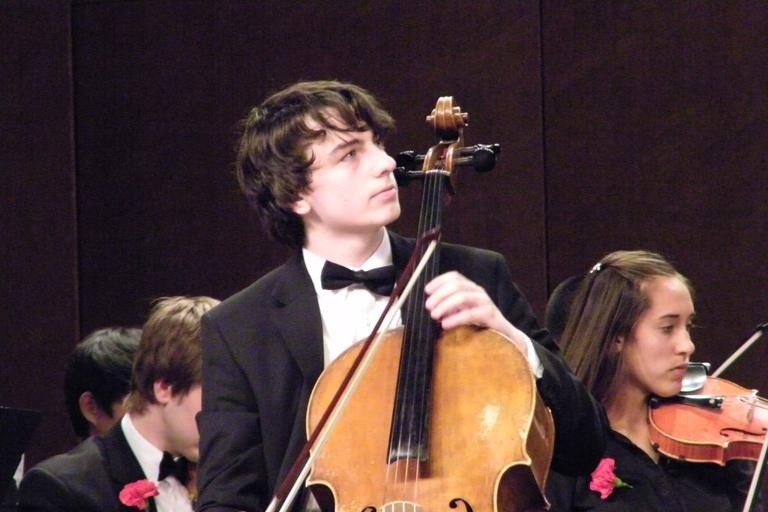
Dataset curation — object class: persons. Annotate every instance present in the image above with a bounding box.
[192,83,603,511]
[545,249,768,511]
[11,291,228,511]
[61,319,143,445]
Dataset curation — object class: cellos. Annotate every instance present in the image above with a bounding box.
[305,96,556,512]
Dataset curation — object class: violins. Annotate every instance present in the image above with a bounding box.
[648,376,768,467]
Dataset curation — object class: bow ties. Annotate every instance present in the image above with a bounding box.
[320,259,399,298]
[157,450,192,488]
